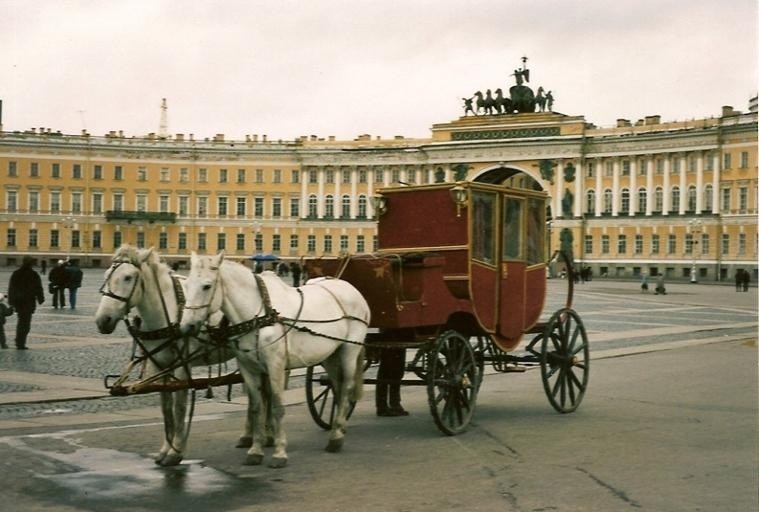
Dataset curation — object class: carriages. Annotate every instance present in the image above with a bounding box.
[91,177,591,469]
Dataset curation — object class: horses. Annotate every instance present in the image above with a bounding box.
[474,91,490,114]
[482,89,500,115]
[494,88,511,113]
[94,241,277,468]
[533,85,546,113]
[178,249,372,469]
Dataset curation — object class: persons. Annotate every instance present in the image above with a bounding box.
[735,269,742,292]
[655,272,664,295]
[743,270,750,291]
[0,293,14,349]
[513,68,525,86]
[640,274,648,293]
[256,261,301,287]
[461,97,477,117]
[546,90,554,112]
[38,258,83,309]
[375,344,410,417]
[9,254,45,350]
[562,265,590,285]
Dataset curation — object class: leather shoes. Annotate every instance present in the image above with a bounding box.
[392,405,409,416]
[377,407,400,417]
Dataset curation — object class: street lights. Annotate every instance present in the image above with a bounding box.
[685,217,704,283]
